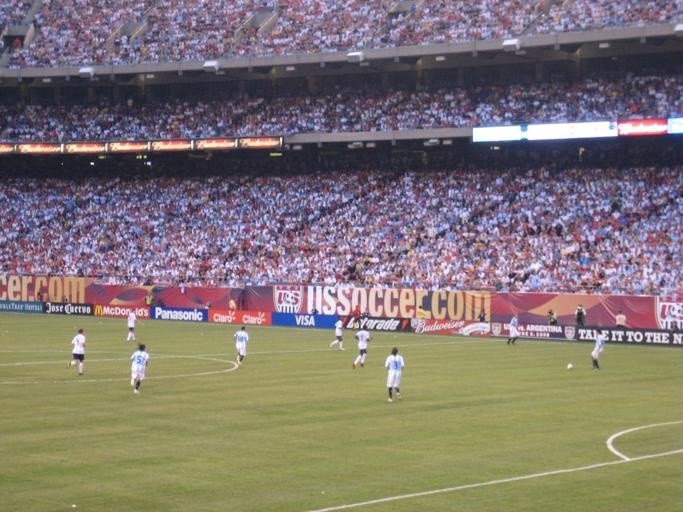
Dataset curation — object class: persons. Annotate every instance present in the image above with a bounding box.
[547,309,558,325]
[574,303,588,327]
[475,308,487,321]
[0,1,682,313]
[232,326,248,361]
[126,308,135,342]
[383,347,404,402]
[589,327,603,371]
[351,325,371,370]
[130,344,149,393]
[65,328,86,376]
[328,316,344,351]
[614,310,627,328]
[507,314,520,345]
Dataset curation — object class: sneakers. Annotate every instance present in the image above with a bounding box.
[68,360,71,367]
[134,389,139,393]
[353,362,364,368]
[236,354,242,365]
[388,393,401,402]
[330,343,345,351]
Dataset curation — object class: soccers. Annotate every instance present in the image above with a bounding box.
[568,363,572,369]
